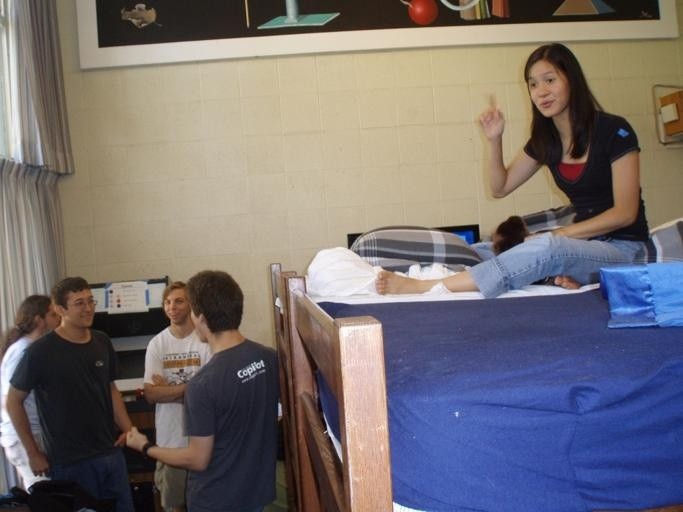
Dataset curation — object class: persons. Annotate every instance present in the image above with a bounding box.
[143,282,213,512]
[5,277,136,512]
[0,295,61,495]
[376,43,650,300]
[126,269,279,512]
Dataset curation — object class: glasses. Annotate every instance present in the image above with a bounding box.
[67,298,97,307]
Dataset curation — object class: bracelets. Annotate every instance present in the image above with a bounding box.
[142,441,159,462]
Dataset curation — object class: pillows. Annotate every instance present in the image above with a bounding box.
[350,226,484,274]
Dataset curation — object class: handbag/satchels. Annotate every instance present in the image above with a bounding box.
[27,480,81,512]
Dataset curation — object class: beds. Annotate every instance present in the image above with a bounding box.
[283,278,682,512]
[270,262,635,511]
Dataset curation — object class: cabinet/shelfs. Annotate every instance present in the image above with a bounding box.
[86,274,173,512]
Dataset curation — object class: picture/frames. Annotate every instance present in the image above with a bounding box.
[75,0,680,71]
